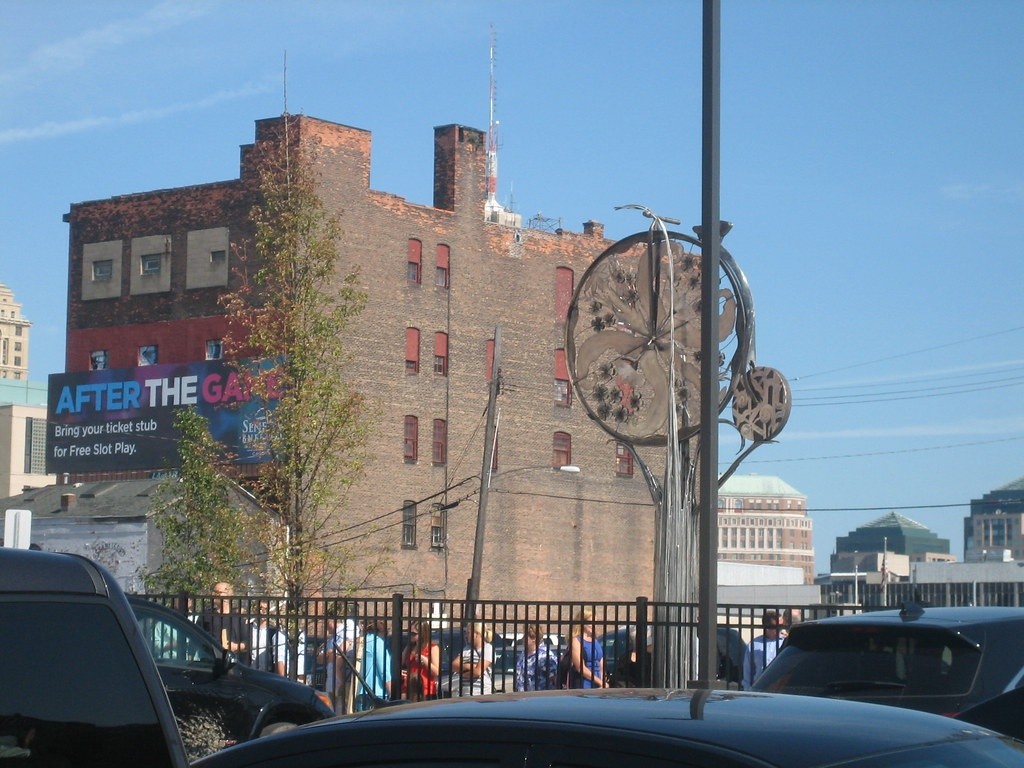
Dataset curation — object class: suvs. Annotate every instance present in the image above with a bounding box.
[748,604,1024,740]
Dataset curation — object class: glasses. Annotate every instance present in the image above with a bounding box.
[411,632,419,637]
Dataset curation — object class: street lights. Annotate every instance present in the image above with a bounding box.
[460,464,580,627]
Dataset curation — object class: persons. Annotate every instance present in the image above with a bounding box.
[152,582,656,717]
[744,608,801,692]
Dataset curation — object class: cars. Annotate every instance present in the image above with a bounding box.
[125,591,335,747]
[186,686,1024,768]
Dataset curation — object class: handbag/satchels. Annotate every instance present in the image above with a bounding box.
[560,642,581,689]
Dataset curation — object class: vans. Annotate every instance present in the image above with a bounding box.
[1,546,189,768]
